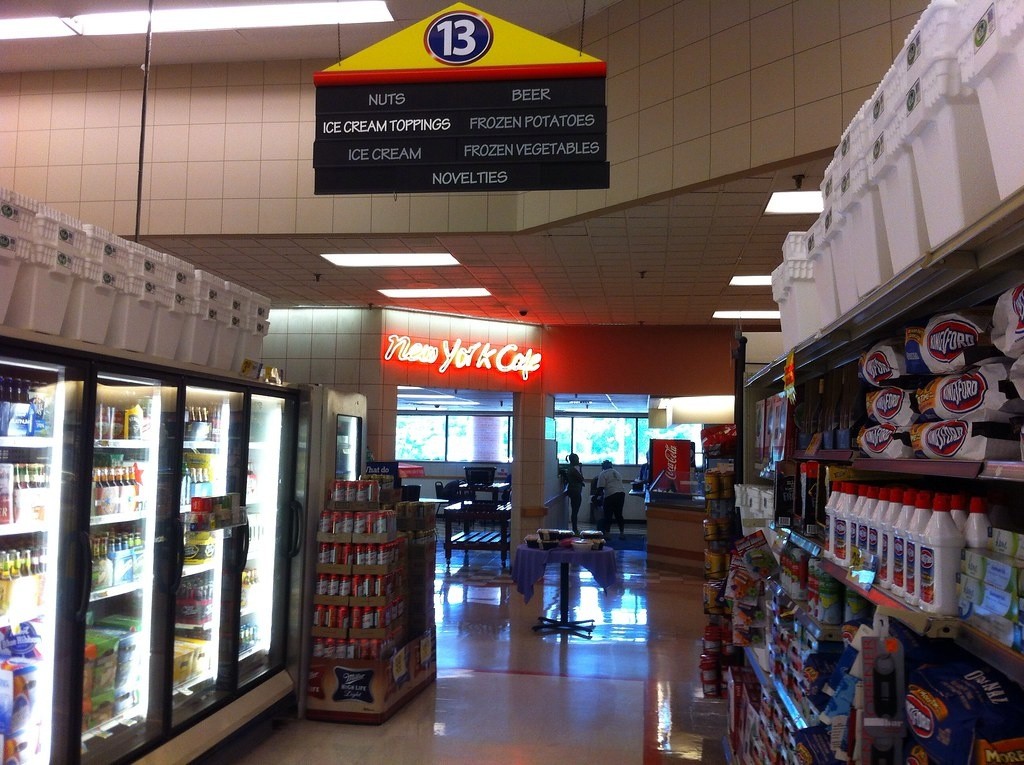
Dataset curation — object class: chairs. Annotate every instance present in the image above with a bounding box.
[435,481,445,516]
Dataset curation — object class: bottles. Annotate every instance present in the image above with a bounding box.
[777,480,992,626]
[0,367,265,662]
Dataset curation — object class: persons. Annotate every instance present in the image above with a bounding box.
[440,479,466,500]
[592,461,625,540]
[591,498,611,540]
[565,453,586,533]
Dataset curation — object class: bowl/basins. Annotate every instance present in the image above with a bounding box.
[523,528,606,551]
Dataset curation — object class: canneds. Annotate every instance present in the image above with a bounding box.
[703,516,734,614]
[870,735,896,765]
[872,655,898,720]
[313,480,437,662]
[704,472,732,499]
[779,541,876,626]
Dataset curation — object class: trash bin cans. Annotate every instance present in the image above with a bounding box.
[865,64,931,275]
[206,281,251,371]
[893,0,1001,249]
[770,263,799,352]
[1,186,39,326]
[3,205,86,336]
[834,98,894,299]
[143,253,195,361]
[781,231,823,343]
[60,223,126,345]
[104,241,163,353]
[174,269,225,367]
[230,291,270,374]
[802,219,843,328]
[938,0,1024,200]
[819,157,861,315]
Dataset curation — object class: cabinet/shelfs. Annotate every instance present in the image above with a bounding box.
[730,185,1024,765]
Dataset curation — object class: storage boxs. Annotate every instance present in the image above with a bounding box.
[956,527,1024,655]
[770,0,1024,356]
[725,665,807,765]
[463,467,497,485]
[754,385,798,463]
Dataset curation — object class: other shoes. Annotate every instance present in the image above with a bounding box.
[574,531,582,538]
[605,532,612,540]
[619,532,625,540]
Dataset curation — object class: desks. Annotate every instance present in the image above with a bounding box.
[459,482,511,510]
[444,501,511,569]
[510,544,617,636]
